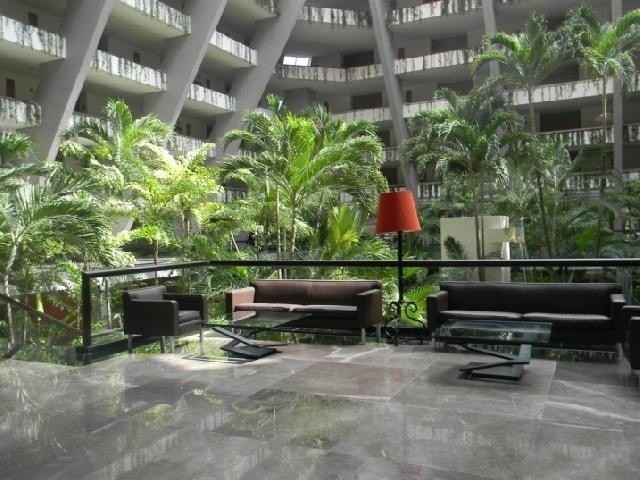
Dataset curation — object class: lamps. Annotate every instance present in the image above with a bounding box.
[375,190,428,347]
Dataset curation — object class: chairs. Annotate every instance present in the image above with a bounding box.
[122,285,208,353]
